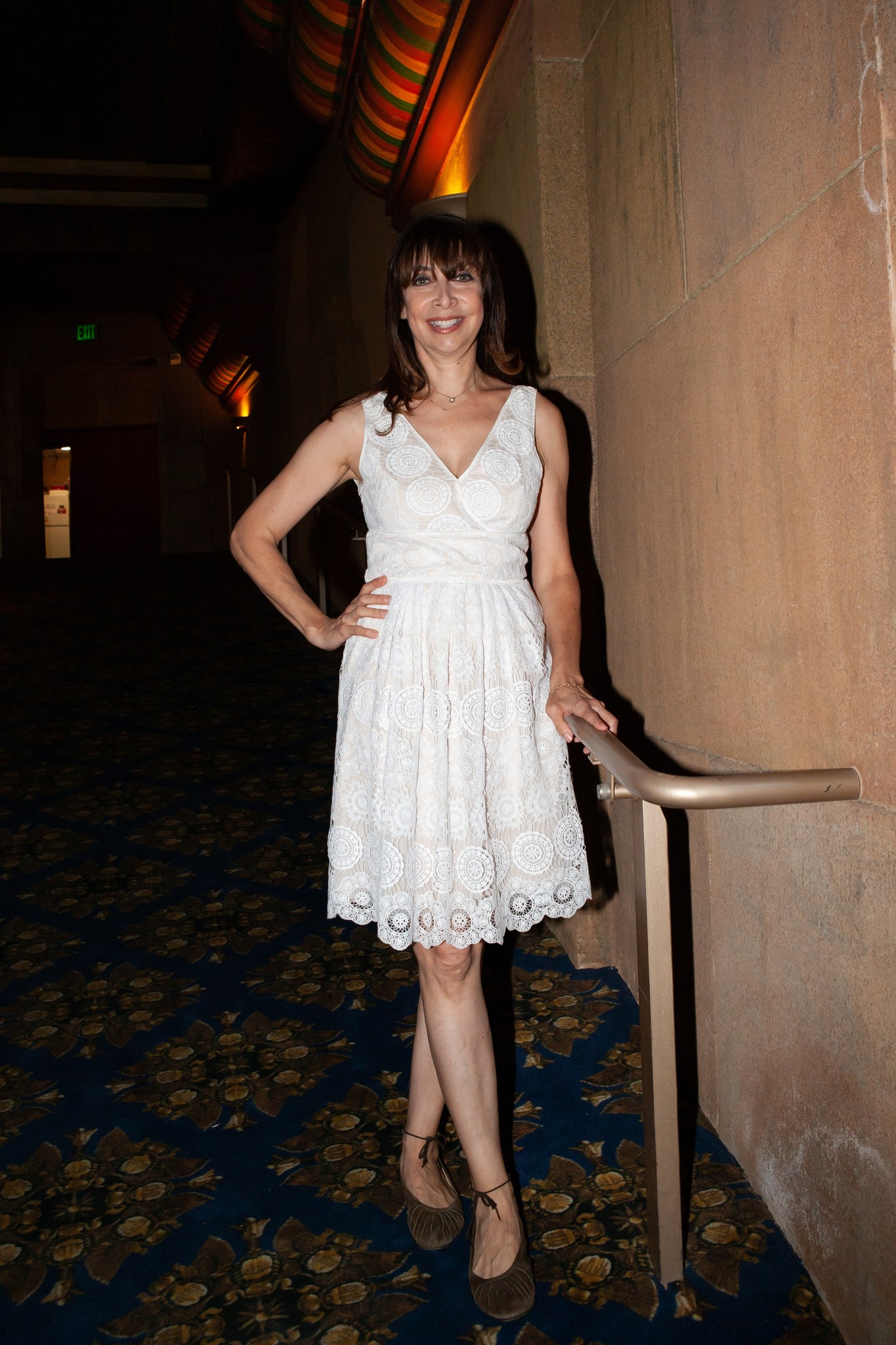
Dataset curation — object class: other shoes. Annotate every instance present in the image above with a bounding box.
[467,1174,536,1320]
[400,1128,465,1250]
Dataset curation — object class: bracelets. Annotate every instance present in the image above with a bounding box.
[549,683,587,693]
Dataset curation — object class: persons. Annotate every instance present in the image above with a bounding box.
[227,216,619,1324]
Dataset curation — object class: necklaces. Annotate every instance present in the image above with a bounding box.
[421,366,484,410]
